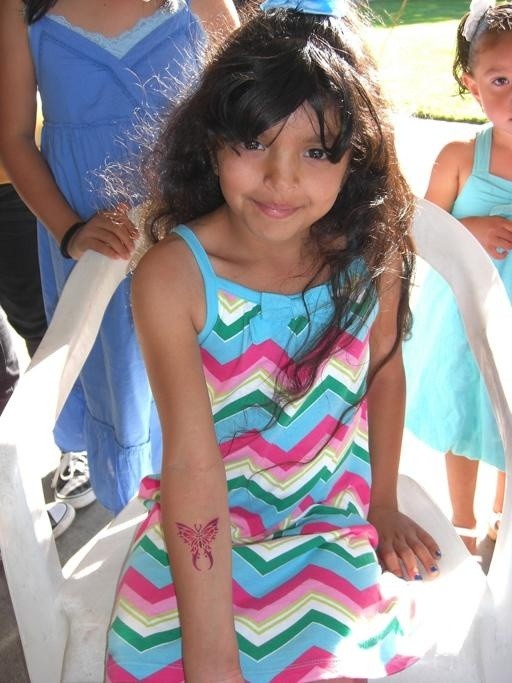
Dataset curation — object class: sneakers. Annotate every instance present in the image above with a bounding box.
[52,444,106,508]
[45,503,75,540]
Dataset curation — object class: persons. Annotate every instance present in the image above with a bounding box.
[96,7,445,682]
[419,0,512,556]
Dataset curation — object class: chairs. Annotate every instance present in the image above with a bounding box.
[1,186,512,680]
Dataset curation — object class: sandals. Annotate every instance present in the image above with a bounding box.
[451,508,501,571]
